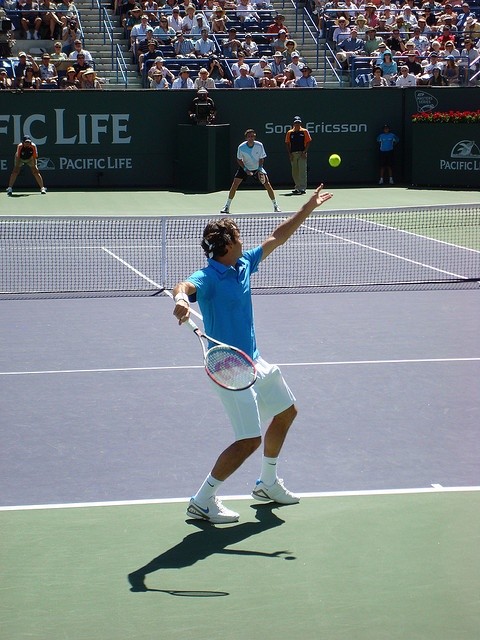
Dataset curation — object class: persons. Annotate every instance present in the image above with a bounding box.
[421,0,443,15]
[352,14,371,39]
[314,0,328,39]
[273,29,289,52]
[19,66,39,89]
[148,56,175,83]
[130,15,153,64]
[62,15,82,54]
[221,28,243,58]
[234,64,256,88]
[160,0,179,19]
[57,0,78,28]
[436,24,455,50]
[461,38,479,74]
[362,2,381,32]
[396,65,416,87]
[441,40,460,63]
[427,68,447,86]
[285,116,312,194]
[166,7,183,34]
[241,33,260,58]
[0,10,15,40]
[173,30,197,59]
[462,16,480,40]
[380,50,397,86]
[39,0,64,40]
[274,67,295,88]
[421,41,445,67]
[443,55,460,86]
[319,0,340,39]
[202,0,228,21]
[182,4,197,34]
[268,51,286,78]
[219,128,282,213]
[405,27,430,59]
[332,27,364,76]
[0,68,15,89]
[38,53,58,89]
[388,16,408,40]
[436,4,457,25]
[402,50,422,85]
[258,66,278,88]
[195,28,218,59]
[457,33,477,50]
[421,8,435,27]
[6,135,48,196]
[193,68,216,89]
[400,0,420,12]
[359,0,378,15]
[266,14,289,43]
[14,52,39,77]
[211,7,227,34]
[126,6,144,50]
[378,0,398,17]
[188,86,217,126]
[286,51,305,79]
[401,41,422,65]
[379,5,396,32]
[456,2,476,31]
[340,0,359,25]
[421,51,443,85]
[282,39,300,64]
[444,0,463,8]
[59,67,80,90]
[414,16,431,33]
[72,53,93,74]
[189,13,211,34]
[137,27,159,72]
[21,0,42,40]
[369,42,390,67]
[363,27,383,67]
[144,0,160,23]
[50,42,67,59]
[434,15,458,40]
[231,52,250,78]
[153,16,177,45]
[69,39,92,60]
[77,68,101,89]
[171,183,334,523]
[171,66,194,89]
[236,0,263,23]
[150,70,168,88]
[376,124,400,184]
[386,29,405,55]
[121,0,143,28]
[179,0,196,17]
[204,54,232,85]
[333,16,351,44]
[369,67,388,86]
[250,56,269,86]
[295,66,317,87]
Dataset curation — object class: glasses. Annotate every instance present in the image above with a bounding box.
[78,57,84,59]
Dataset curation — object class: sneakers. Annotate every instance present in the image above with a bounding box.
[41,187,47,194]
[379,179,383,184]
[186,495,240,523]
[274,206,281,212]
[250,477,300,504]
[389,180,395,184]
[220,205,229,213]
[6,187,13,195]
[292,190,306,195]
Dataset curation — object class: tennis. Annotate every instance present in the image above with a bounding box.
[329,154,341,168]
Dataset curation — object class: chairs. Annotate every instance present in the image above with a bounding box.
[226,14,272,57]
[354,68,374,85]
[158,43,204,79]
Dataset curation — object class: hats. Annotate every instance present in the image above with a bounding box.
[154,56,166,64]
[463,16,478,27]
[272,51,284,57]
[82,67,96,80]
[355,15,367,25]
[41,52,50,59]
[66,66,76,74]
[239,65,247,71]
[198,68,210,77]
[293,115,302,123]
[185,5,196,14]
[334,16,350,26]
[178,66,192,75]
[405,50,418,56]
[152,70,162,76]
[212,6,226,16]
[23,135,32,142]
[283,39,297,50]
[258,56,268,64]
[381,50,393,60]
[18,51,26,57]
[404,41,415,49]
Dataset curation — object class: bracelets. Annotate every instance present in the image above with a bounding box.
[243,167,248,172]
[174,292,190,305]
